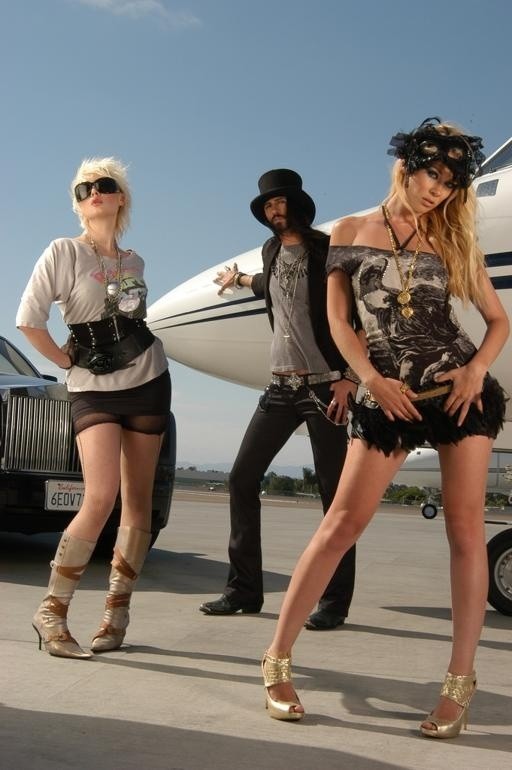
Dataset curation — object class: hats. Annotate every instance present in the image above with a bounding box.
[250,169,315,225]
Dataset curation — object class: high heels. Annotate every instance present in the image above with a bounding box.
[419,670,477,737]
[199,594,263,615]
[261,651,305,719]
[304,609,345,629]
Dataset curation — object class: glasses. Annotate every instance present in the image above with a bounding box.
[74,177,124,202]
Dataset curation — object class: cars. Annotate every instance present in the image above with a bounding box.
[0,337,178,567]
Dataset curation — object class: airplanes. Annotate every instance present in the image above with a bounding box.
[145,134,511,617]
[388,441,512,519]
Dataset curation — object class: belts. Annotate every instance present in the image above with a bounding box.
[271,371,340,390]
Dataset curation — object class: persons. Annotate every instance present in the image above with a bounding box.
[197,167,370,629]
[14,158,172,658]
[262,116,509,739]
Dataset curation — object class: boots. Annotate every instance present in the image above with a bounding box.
[91,526,152,653]
[32,530,98,659]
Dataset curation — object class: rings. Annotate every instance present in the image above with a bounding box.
[399,383,411,393]
[331,399,336,405]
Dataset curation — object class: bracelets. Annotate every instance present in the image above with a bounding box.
[342,367,361,385]
[63,348,75,372]
[233,273,245,289]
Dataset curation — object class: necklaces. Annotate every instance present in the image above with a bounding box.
[277,243,303,342]
[385,208,421,253]
[88,237,122,300]
[377,205,428,321]
